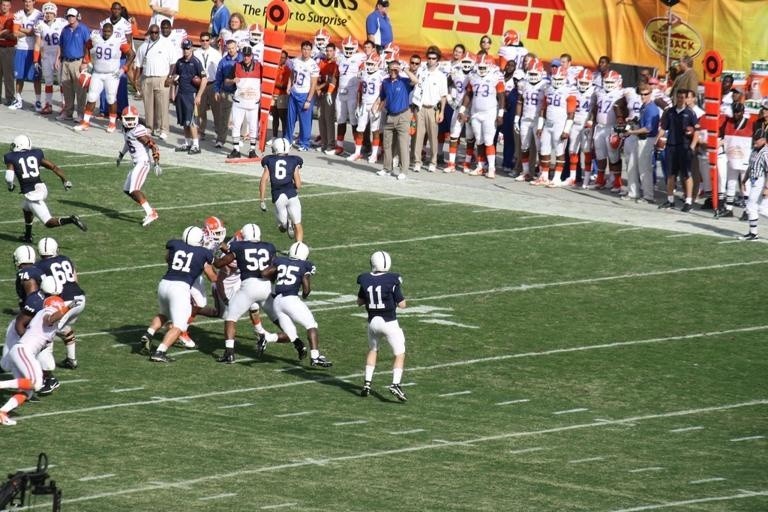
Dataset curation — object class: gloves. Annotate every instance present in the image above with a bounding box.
[64,180,72,191]
[8,184,15,191]
[326,93,333,105]
[260,201,267,211]
[34,63,42,73]
[113,69,124,79]
[354,106,360,117]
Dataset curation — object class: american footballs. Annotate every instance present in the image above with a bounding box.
[192,76,202,88]
[657,136,667,151]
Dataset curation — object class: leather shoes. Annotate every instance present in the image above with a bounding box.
[387,109,408,116]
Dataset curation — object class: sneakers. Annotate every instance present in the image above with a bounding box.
[299,147,309,152]
[414,163,421,172]
[398,173,406,180]
[530,177,549,185]
[188,146,201,154]
[256,334,267,357]
[636,198,655,203]
[71,215,88,231]
[174,333,195,348]
[715,210,732,217]
[376,170,391,176]
[73,115,82,122]
[390,384,407,401]
[610,182,623,193]
[443,165,456,173]
[287,218,295,239]
[620,196,637,201]
[34,101,42,112]
[547,180,560,186]
[74,121,89,130]
[106,122,116,132]
[463,165,471,173]
[215,141,222,147]
[9,99,22,109]
[658,201,674,208]
[0,412,16,426]
[56,113,72,120]
[561,178,576,187]
[587,183,606,190]
[139,333,153,355]
[175,144,191,151]
[346,153,360,161]
[325,147,344,155]
[65,359,77,368]
[19,236,32,242]
[151,350,175,362]
[142,212,158,226]
[488,170,496,178]
[508,171,519,177]
[216,354,234,362]
[40,105,52,114]
[249,150,258,157]
[428,164,436,172]
[368,156,377,163]
[361,386,371,396]
[227,149,241,159]
[470,169,486,176]
[737,232,759,241]
[515,173,532,181]
[159,134,168,141]
[681,203,694,212]
[40,377,59,394]
[311,355,332,367]
[297,344,307,359]
[701,198,713,209]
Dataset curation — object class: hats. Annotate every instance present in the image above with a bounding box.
[731,87,743,92]
[753,128,768,139]
[241,47,252,54]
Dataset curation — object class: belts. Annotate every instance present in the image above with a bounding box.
[207,80,216,85]
[65,56,83,61]
[645,134,653,136]
[423,105,433,108]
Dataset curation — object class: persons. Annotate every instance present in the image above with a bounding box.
[4,133,88,245]
[0,237,88,426]
[1,0,265,164]
[355,248,410,403]
[736,127,768,243]
[268,1,758,223]
[259,138,306,243]
[139,214,331,368]
[115,106,166,228]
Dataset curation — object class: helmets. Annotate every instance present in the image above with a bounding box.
[527,59,543,83]
[121,106,139,128]
[384,42,400,62]
[205,216,225,242]
[603,69,623,91]
[182,226,204,246]
[10,134,31,152]
[461,51,476,71]
[41,275,63,295]
[314,28,330,48]
[504,30,519,45]
[249,24,264,43]
[576,67,593,91]
[272,137,290,155]
[366,52,381,73]
[241,223,261,242]
[371,250,391,272]
[44,296,69,320]
[427,46,441,57]
[66,8,78,17]
[552,67,566,89]
[476,54,495,77]
[342,35,358,57]
[289,241,309,260]
[609,131,624,149]
[38,237,58,256]
[79,72,91,88]
[182,39,192,47]
[13,245,36,266]
[42,2,57,15]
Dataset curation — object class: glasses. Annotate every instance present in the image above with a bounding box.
[428,58,436,59]
[640,93,649,95]
[201,39,210,42]
[411,62,419,65]
[150,31,158,33]
[244,54,251,56]
[183,47,190,49]
